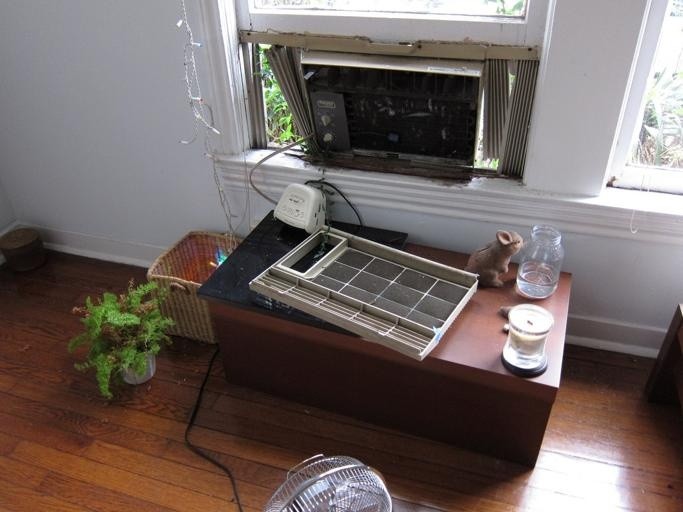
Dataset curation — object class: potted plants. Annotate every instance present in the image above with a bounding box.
[67,276,177,402]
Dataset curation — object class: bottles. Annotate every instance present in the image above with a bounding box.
[515,224,564,300]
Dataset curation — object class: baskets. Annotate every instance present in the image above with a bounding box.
[147,231,244,344]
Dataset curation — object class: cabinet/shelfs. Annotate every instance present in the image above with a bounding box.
[206,241,572,468]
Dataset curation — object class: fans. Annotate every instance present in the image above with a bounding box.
[265,453,393,512]
